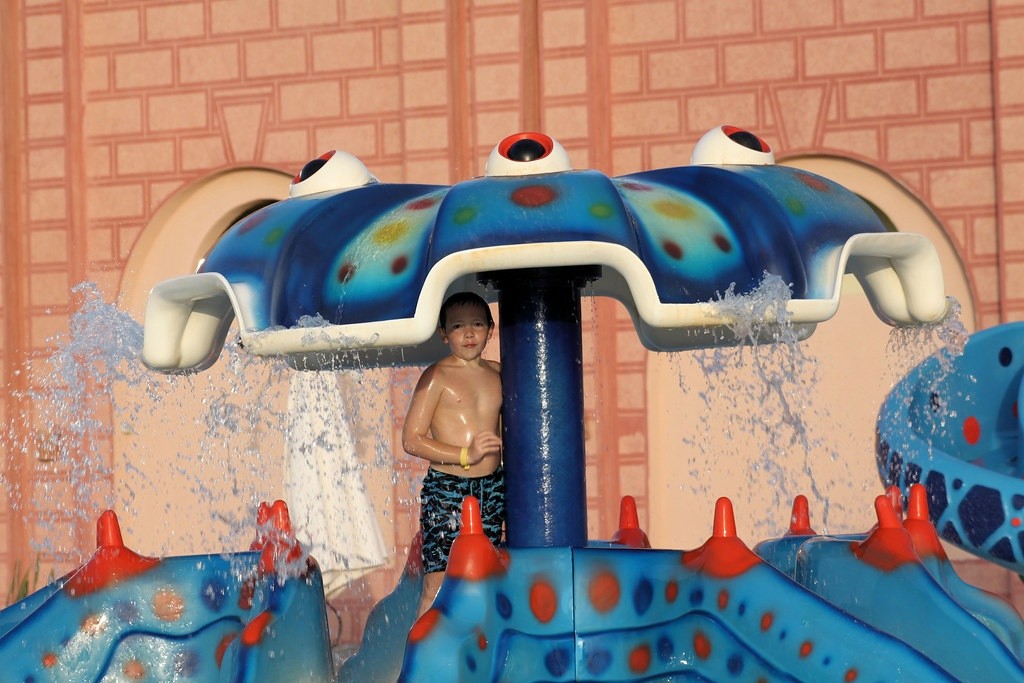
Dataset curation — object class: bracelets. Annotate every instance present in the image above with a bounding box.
[460,447,470,470]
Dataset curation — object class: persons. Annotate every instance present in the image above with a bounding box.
[402,292,504,620]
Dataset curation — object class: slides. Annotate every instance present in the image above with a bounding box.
[877,321,1024,576]
